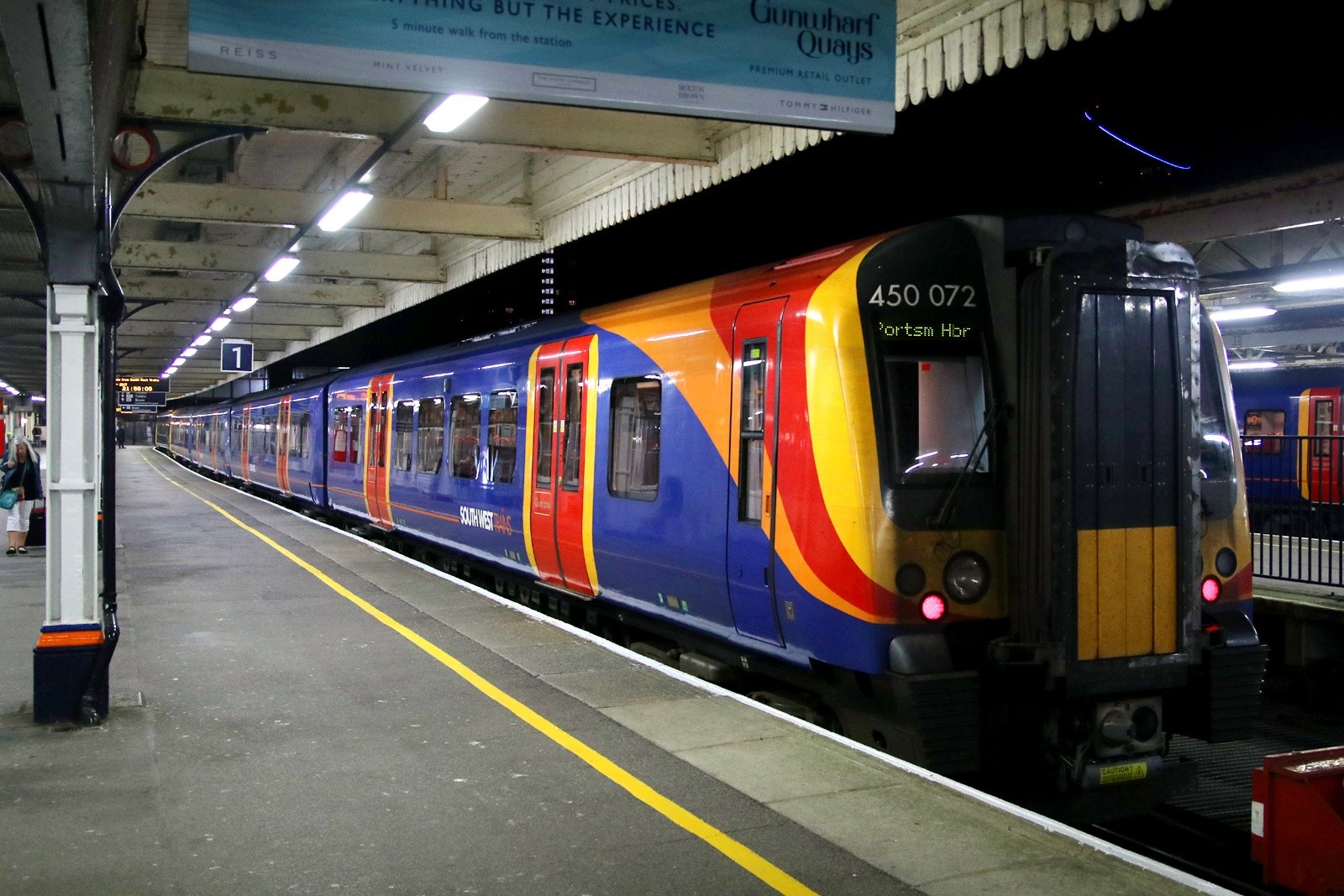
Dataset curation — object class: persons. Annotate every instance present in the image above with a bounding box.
[116,424,126,449]
[0,436,44,554]
[32,423,42,448]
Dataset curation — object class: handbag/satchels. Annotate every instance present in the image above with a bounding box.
[0,489,21,509]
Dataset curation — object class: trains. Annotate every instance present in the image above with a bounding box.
[151,210,1274,790]
[1196,301,1343,538]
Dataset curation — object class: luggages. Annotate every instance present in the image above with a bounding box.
[25,497,46,547]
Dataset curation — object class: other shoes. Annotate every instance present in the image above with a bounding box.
[17,546,27,554]
[6,546,16,555]
